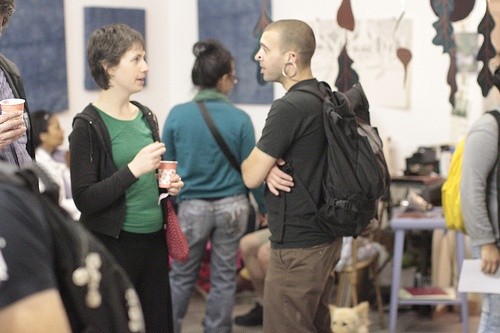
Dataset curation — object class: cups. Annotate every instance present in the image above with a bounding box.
[158,161,178,189]
[0,99,26,128]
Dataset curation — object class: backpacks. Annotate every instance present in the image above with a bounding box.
[276,81,387,240]
[14,163,147,333]
[441,110,500,232]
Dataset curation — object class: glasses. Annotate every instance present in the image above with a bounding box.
[228,72,240,86]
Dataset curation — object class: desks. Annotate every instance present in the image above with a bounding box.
[389,175,444,205]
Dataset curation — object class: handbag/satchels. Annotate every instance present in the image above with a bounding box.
[163,193,190,264]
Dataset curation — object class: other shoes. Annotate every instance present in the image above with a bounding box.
[235,302,263,327]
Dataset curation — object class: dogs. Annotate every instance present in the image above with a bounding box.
[327,301,370,333]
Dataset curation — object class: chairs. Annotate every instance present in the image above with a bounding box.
[333,235,384,331]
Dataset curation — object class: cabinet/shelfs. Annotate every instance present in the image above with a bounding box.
[390,219,468,333]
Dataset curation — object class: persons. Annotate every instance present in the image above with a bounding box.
[69,23,184,333]
[160,46,268,332]
[240,20,342,333]
[235,227,271,327]
[0,0,77,331]
[459,108,500,332]
